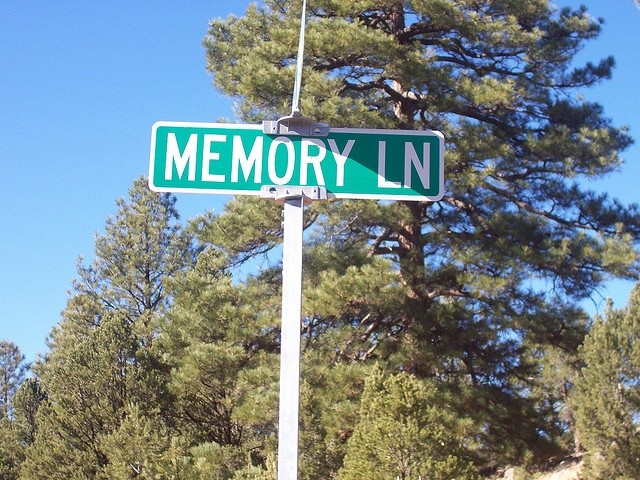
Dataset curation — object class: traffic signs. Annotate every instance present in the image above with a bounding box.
[149,120,446,201]
